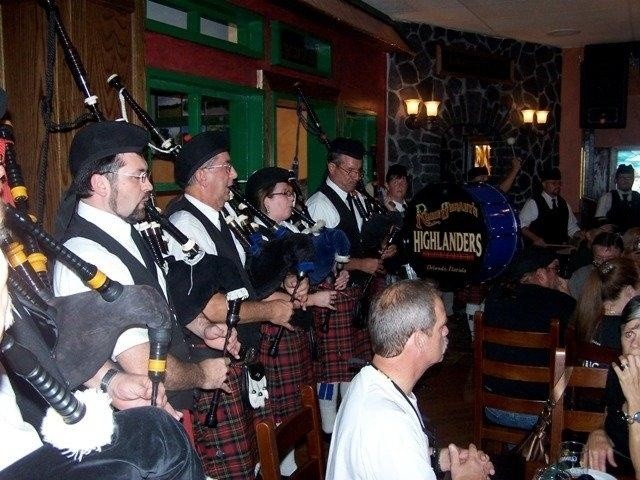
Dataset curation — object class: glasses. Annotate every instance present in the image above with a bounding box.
[202,160,234,175]
[100,170,152,182]
[332,162,360,179]
[268,191,294,197]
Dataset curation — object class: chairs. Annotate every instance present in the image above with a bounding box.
[473,312,559,479]
[561,324,623,459]
[256,385,324,480]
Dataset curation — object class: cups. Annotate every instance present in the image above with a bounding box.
[557,441,588,479]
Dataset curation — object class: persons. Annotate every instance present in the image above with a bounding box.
[302,136,399,445]
[0,152,205,479]
[326,280,495,480]
[53,120,241,455]
[164,130,310,480]
[386,154,639,453]
[246,166,350,480]
[580,295,640,480]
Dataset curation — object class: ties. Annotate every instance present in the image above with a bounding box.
[346,193,358,234]
[622,194,628,206]
[219,211,241,263]
[552,198,556,209]
[131,224,157,283]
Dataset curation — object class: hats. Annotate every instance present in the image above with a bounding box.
[174,130,228,185]
[467,167,488,181]
[330,137,366,158]
[541,167,561,181]
[520,247,557,272]
[69,121,149,173]
[244,168,289,191]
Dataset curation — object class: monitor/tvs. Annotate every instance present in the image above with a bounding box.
[608,145,640,194]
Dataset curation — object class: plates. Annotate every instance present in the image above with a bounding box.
[565,468,617,480]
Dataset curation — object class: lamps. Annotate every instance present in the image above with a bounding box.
[404,99,441,132]
[518,109,551,130]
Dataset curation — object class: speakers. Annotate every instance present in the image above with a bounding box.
[581,41,629,129]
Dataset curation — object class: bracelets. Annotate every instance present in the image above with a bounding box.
[431,447,442,476]
[100,367,122,395]
